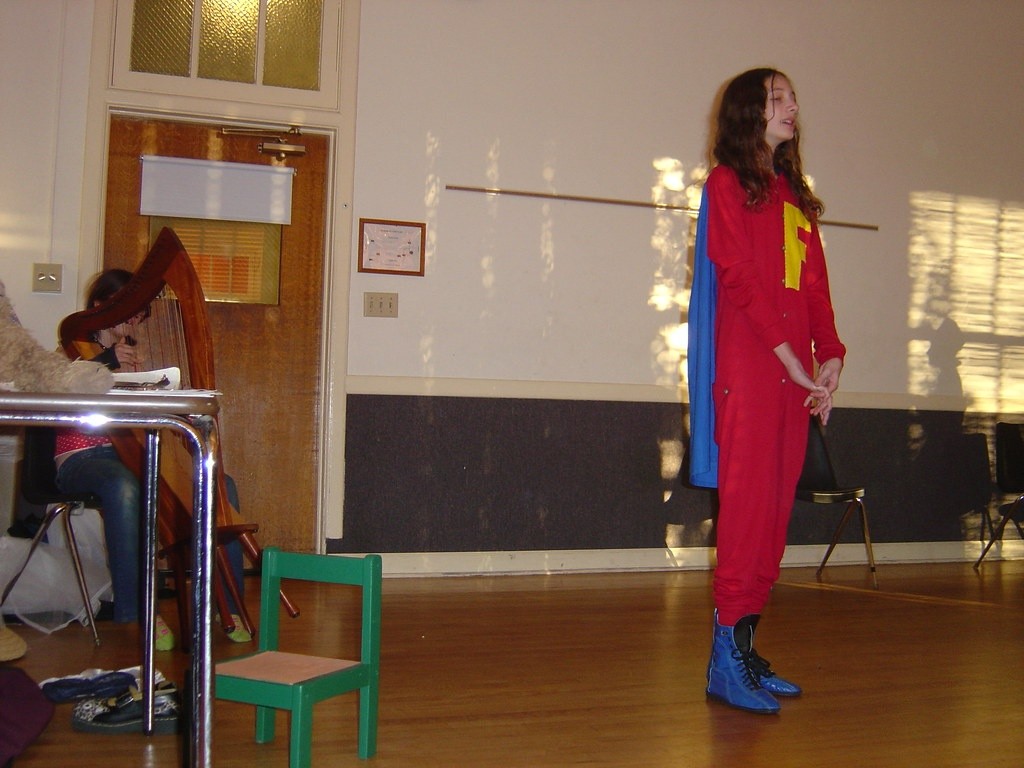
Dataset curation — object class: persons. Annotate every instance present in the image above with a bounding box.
[47,268,252,651]
[685,67,846,715]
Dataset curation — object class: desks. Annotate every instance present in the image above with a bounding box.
[0,393,221,768]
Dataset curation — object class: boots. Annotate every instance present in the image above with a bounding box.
[749,648,803,697]
[705,608,783,714]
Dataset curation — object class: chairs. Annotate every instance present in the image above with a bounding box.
[215,545,383,768]
[1,425,103,648]
[974,421,1024,572]
[794,415,881,590]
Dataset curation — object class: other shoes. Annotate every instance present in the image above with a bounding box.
[71,679,182,734]
[37,665,165,705]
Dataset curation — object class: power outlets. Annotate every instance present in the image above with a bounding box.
[363,291,398,318]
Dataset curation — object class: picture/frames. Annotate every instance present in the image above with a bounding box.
[357,218,427,277]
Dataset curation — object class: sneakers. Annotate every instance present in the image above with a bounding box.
[154,615,175,651]
[216,612,252,643]
